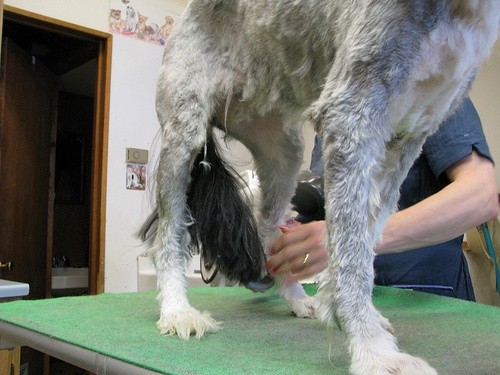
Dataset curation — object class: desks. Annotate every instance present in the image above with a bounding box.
[0,275,500,375]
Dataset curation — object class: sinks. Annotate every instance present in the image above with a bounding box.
[50,266,89,290]
[0,277,30,299]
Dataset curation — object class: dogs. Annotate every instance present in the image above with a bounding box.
[135,1,500,375]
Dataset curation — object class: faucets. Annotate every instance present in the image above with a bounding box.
[0,260,12,272]
[52,254,72,268]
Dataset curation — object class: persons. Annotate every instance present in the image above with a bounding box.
[264,97,500,302]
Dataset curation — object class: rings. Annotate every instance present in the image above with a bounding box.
[302,252,313,269]
